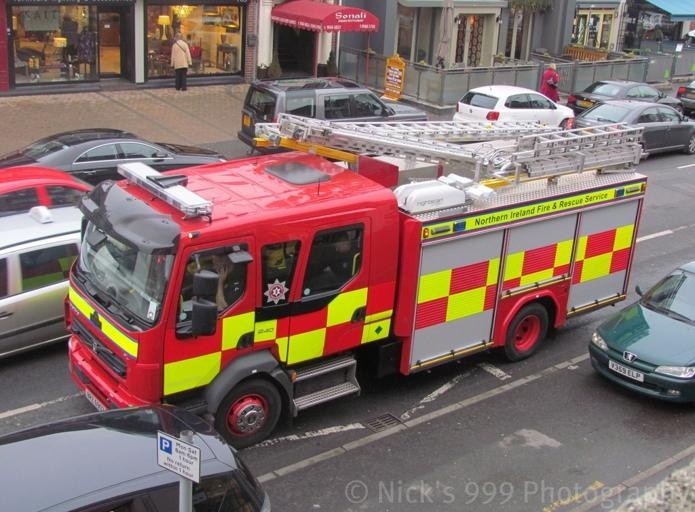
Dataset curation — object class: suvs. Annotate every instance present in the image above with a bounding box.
[234,75,432,155]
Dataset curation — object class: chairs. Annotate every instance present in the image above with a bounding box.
[14,41,29,83]
[146,48,205,77]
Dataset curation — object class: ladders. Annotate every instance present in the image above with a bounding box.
[252,112,649,178]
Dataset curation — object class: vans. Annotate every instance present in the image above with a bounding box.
[0,397,279,512]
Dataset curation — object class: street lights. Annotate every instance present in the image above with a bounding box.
[666,37,687,78]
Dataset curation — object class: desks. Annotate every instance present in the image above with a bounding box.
[216,45,237,71]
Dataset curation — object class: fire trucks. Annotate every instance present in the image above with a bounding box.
[59,107,649,449]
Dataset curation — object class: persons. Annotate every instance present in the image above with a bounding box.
[322,232,352,282]
[170,32,192,91]
[538,63,560,102]
[211,255,244,311]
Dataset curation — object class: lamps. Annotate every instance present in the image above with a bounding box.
[51,37,67,67]
[158,16,170,41]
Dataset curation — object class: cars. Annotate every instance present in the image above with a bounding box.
[585,258,695,412]
[453,75,695,165]
